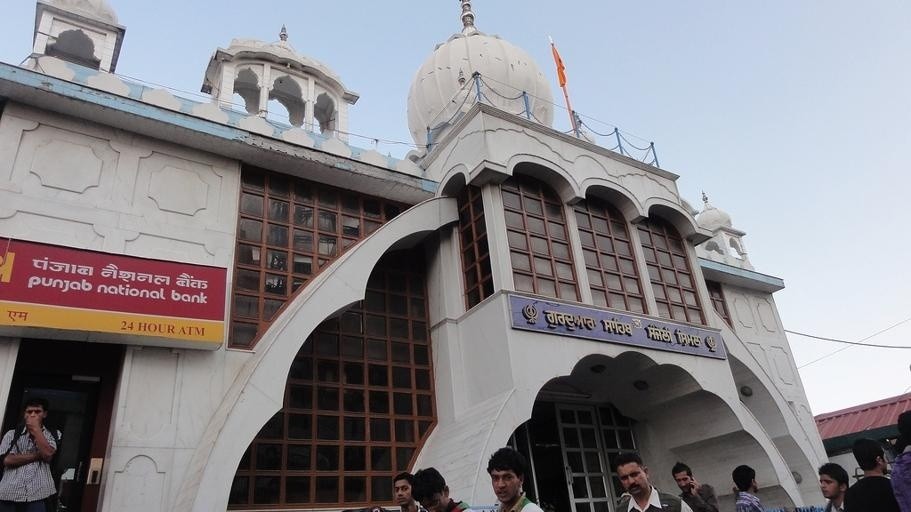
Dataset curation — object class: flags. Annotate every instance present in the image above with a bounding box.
[552,45,568,88]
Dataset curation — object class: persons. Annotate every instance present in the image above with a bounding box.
[732,463,765,512]
[795,462,851,511]
[890,409,911,511]
[1,393,58,511]
[411,467,475,512]
[672,460,720,511]
[612,451,695,511]
[844,438,902,511]
[394,472,429,511]
[487,447,546,512]
